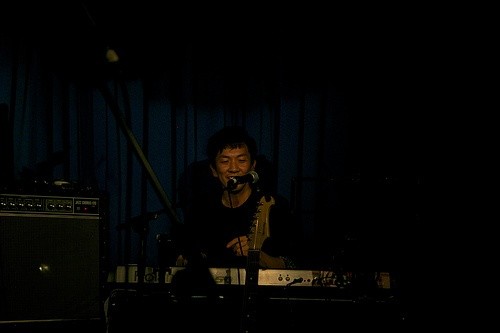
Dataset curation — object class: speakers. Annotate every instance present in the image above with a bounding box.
[0,191,102,325]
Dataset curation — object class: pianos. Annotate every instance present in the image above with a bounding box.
[115,263,390,303]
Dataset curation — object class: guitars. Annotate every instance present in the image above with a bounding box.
[242,196,276,332]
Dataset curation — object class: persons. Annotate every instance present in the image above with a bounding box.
[183,126,306,272]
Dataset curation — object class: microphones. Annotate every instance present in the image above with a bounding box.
[227,170,259,186]
